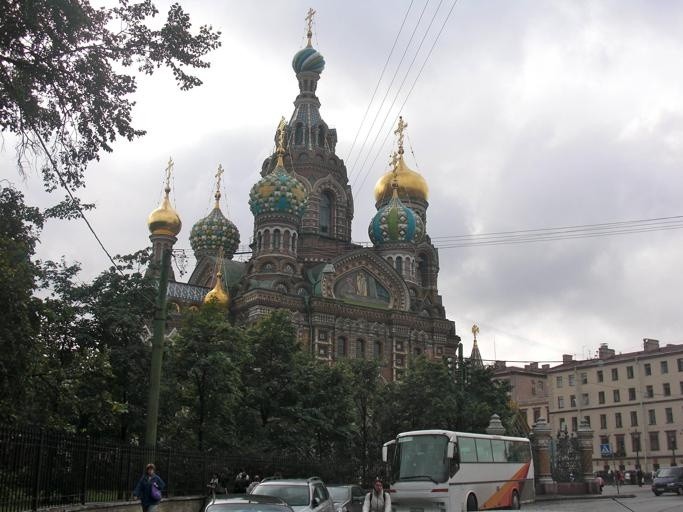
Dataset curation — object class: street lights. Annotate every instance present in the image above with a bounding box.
[630,427,644,489]
[668,433,676,468]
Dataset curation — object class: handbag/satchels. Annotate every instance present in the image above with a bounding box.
[149,481,161,500]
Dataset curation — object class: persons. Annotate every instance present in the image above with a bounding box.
[597,468,656,495]
[361,479,392,512]
[133,462,166,512]
[206,469,262,496]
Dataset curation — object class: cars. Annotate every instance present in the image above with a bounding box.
[204,475,367,512]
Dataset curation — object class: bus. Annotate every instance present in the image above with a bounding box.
[380,430,536,511]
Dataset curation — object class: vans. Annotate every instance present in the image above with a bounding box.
[650,466,682,497]
